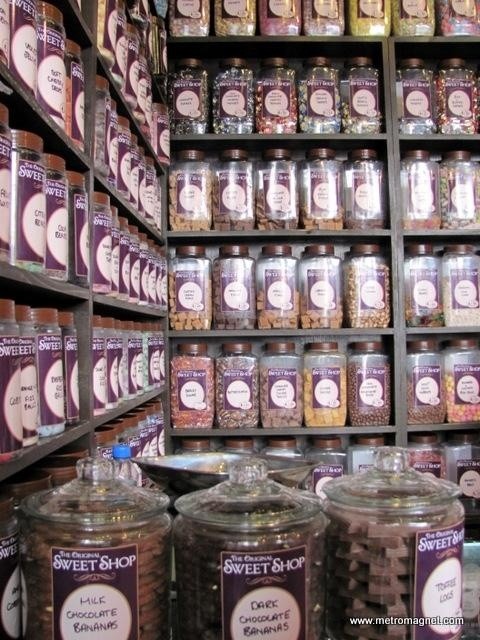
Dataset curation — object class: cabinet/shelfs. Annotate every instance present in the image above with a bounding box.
[0,0,171,480]
[172,29,479,449]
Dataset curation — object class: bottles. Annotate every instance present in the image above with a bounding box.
[169,461,330,640]
[0,495,28,640]
[323,446,465,639]
[1,3,480,515]
[19,448,170,640]
